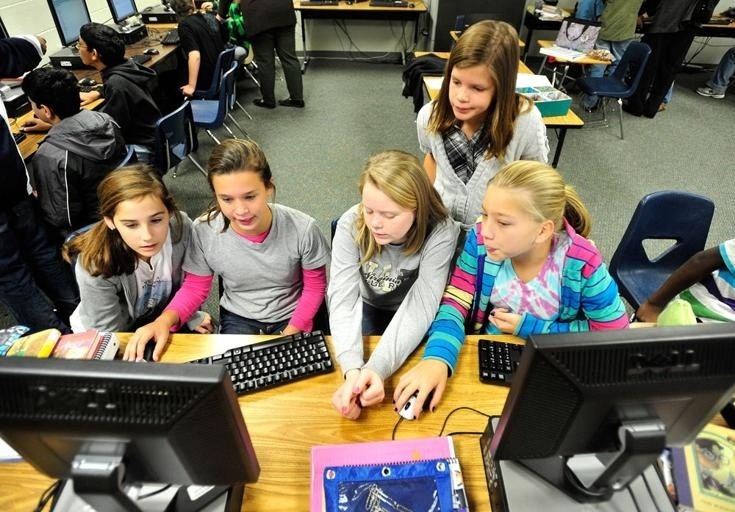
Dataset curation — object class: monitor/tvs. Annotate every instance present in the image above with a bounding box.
[107,0,138,24]
[47,0,92,46]
[491,320,735,512]
[0,353,261,512]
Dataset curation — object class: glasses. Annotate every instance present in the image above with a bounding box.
[76,41,88,49]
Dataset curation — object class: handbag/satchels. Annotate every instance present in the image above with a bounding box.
[556,17,602,53]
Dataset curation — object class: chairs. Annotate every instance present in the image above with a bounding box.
[112,144,142,170]
[608,190,715,322]
[575,40,652,140]
[63,221,101,268]
[145,97,208,178]
[189,60,239,147]
[192,47,253,128]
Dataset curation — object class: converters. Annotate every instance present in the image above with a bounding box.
[421,29,429,37]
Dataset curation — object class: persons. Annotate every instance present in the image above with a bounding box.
[579,0,643,115]
[19,67,137,230]
[392,159,631,422]
[697,46,735,98]
[216,0,306,109]
[636,236,735,329]
[61,163,220,337]
[0,93,81,335]
[415,19,550,239]
[75,22,162,171]
[325,149,466,423]
[574,0,604,26]
[122,138,330,363]
[163,0,219,102]
[621,0,720,119]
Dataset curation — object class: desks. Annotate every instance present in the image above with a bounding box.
[414,50,584,168]
[0,325,735,512]
[2,20,183,169]
[292,0,428,75]
[535,39,611,93]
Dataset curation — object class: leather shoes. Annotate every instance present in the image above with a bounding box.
[253,98,275,108]
[279,98,304,108]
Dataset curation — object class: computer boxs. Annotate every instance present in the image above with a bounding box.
[142,4,179,23]
[0,81,32,116]
[49,47,96,70]
[116,25,151,48]
[481,415,677,512]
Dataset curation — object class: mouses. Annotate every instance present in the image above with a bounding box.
[143,48,159,55]
[136,338,160,363]
[19,125,32,131]
[78,77,96,86]
[396,390,435,421]
[347,1,352,5]
[409,3,415,8]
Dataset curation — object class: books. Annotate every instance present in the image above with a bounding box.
[0,322,120,466]
[539,44,586,62]
[690,429,735,510]
[516,85,572,118]
[305,434,469,512]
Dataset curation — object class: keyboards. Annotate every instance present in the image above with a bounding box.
[12,132,27,144]
[369,0,407,7]
[161,29,180,45]
[300,0,338,6]
[478,339,523,387]
[133,55,152,65]
[186,331,336,398]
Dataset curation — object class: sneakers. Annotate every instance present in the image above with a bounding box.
[696,87,724,98]
